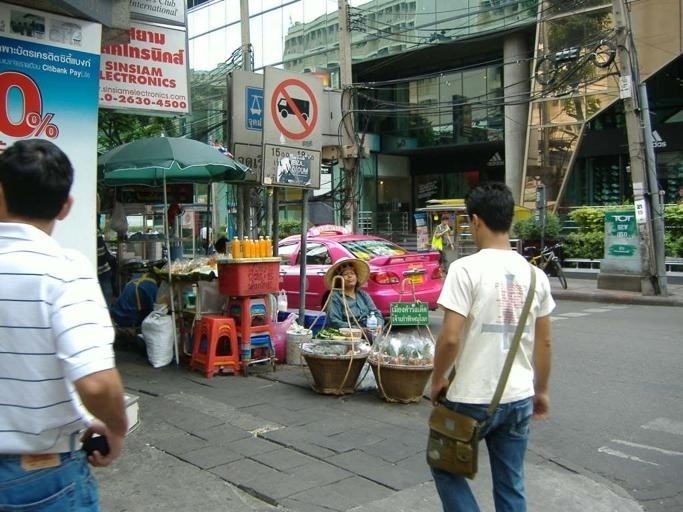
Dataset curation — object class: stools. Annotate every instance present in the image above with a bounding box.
[187,294,276,379]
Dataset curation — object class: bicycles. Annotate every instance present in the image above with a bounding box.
[523,243,567,290]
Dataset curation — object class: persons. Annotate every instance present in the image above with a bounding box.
[0,139,128,512]
[96,210,119,309]
[427,180,558,511]
[430,217,455,271]
[111,271,158,330]
[324,255,385,340]
[198,221,212,249]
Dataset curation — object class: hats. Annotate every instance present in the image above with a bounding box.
[323,256,370,290]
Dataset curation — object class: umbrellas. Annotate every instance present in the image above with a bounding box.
[98,134,250,362]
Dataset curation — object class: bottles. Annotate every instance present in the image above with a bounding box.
[241,234,272,259]
[365,310,376,333]
[276,290,289,312]
[230,236,240,259]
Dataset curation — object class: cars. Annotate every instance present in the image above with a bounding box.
[269,224,443,317]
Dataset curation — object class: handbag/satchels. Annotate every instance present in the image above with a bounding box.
[431,225,443,251]
[425,398,483,482]
